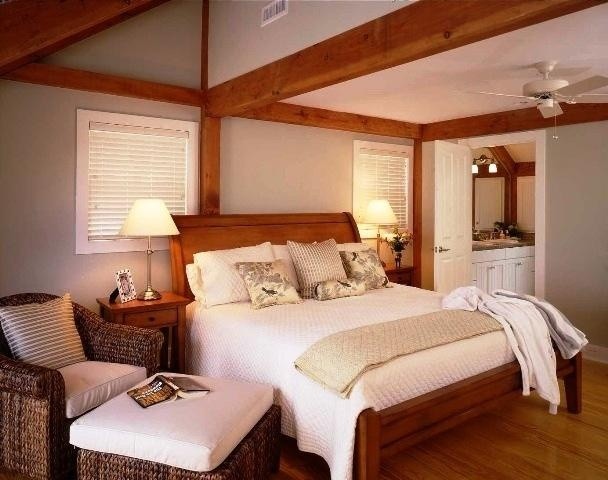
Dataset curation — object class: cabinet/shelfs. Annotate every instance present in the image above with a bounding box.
[504,244,535,298]
[471,249,507,297]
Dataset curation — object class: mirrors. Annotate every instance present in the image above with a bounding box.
[472,147,513,238]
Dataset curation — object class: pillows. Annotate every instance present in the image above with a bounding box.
[341,248,394,293]
[185,260,208,304]
[0,291,89,372]
[235,256,302,311]
[189,242,278,308]
[273,237,371,292]
[285,232,344,297]
[309,274,369,301]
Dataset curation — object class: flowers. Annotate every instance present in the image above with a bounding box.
[376,226,416,256]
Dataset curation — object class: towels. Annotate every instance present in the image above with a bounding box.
[492,286,590,361]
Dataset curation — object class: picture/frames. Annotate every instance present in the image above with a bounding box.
[113,267,139,305]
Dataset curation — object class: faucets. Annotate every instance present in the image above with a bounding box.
[490,231,498,240]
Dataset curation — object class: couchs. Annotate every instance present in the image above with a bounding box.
[0,289,284,480]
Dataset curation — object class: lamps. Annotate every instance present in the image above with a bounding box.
[471,154,484,174]
[481,152,497,174]
[115,196,185,302]
[533,92,557,108]
[356,198,399,258]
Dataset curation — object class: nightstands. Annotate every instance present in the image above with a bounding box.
[375,260,415,288]
[94,289,191,376]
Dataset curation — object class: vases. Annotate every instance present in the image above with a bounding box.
[392,249,404,270]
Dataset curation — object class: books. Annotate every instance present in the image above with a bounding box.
[126,375,210,409]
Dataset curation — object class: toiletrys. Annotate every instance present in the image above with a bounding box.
[505,229,510,238]
[499,229,505,239]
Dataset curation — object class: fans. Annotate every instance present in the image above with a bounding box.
[463,58,607,119]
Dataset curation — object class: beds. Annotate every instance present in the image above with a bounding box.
[165,207,591,480]
